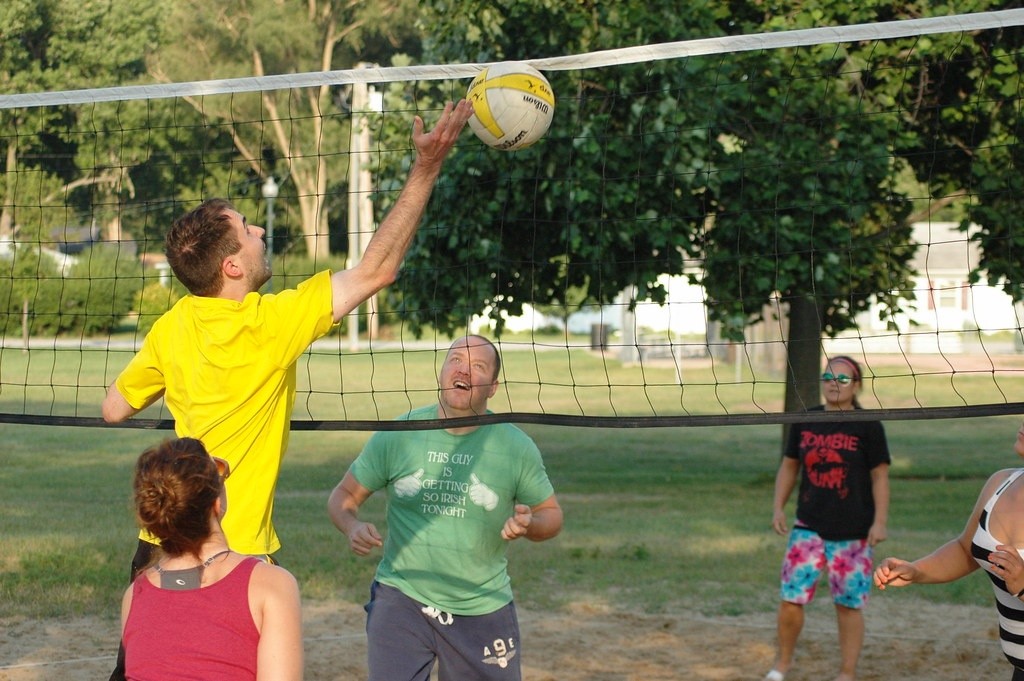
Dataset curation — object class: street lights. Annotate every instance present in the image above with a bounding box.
[261,176,279,295]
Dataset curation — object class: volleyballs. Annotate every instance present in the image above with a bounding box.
[464,61,554,152]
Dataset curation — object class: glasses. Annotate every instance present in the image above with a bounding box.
[211,457,230,506]
[822,373,854,384]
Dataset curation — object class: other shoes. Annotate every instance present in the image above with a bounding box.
[764,657,795,681]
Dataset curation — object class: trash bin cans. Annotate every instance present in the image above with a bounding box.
[591,324,607,351]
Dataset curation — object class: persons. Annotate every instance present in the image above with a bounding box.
[762,356,892,681]
[121,437,304,681]
[101,95,474,681]
[327,334,563,681]
[873,424,1024,681]
